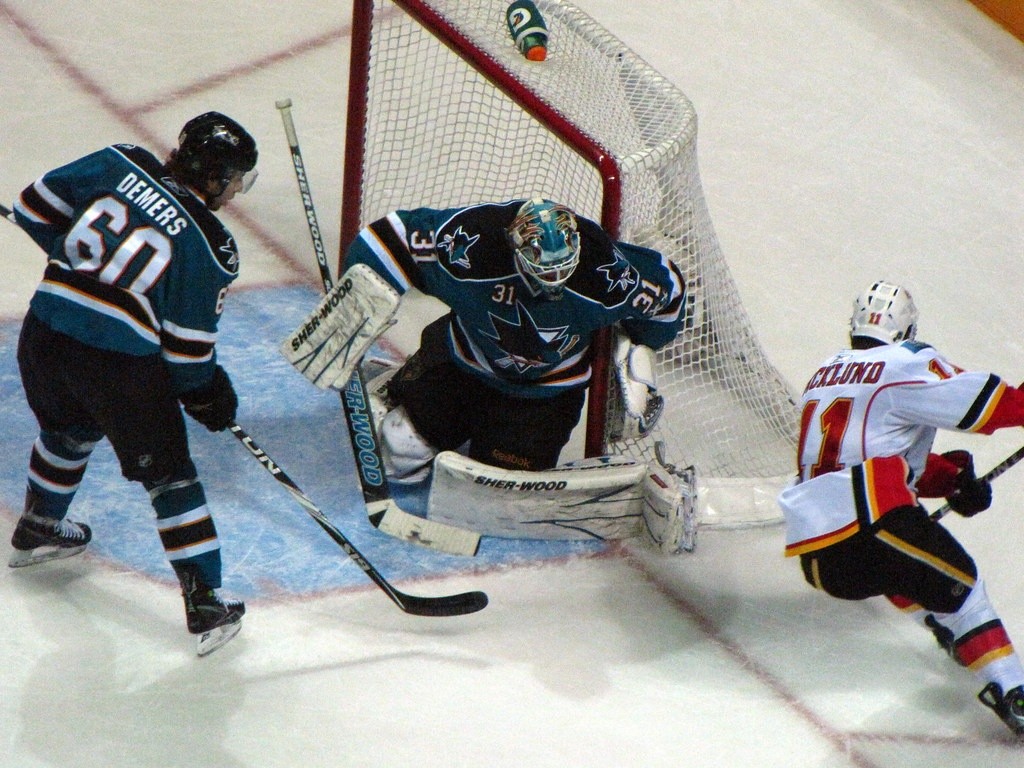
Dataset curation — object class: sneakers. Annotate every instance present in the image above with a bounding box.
[8,485,92,568]
[180,575,246,657]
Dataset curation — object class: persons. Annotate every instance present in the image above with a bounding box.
[7,111,261,656]
[279,197,689,485]
[779,280,1024,737]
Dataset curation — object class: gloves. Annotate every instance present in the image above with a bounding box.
[938,449,993,518]
[178,365,238,433]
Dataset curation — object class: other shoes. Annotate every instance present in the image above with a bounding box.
[977,681,1024,744]
[924,613,965,666]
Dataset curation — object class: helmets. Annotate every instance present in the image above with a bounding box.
[848,280,920,345]
[177,111,258,172]
[504,197,581,287]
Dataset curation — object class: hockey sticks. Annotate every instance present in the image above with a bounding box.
[0,202,490,618]
[926,448,1024,522]
[276,95,484,554]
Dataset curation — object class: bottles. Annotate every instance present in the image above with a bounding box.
[506,0,550,61]
